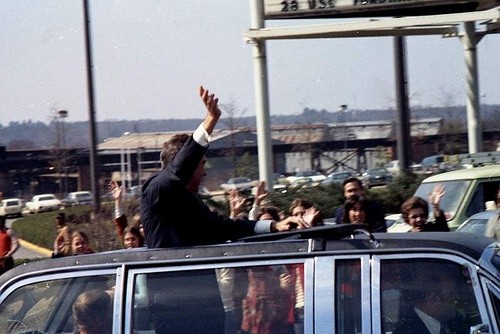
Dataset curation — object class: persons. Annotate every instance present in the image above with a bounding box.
[400,183,451,232]
[342,194,386,233]
[0,214,21,274]
[70,230,99,254]
[394,274,467,334]
[72,291,113,334]
[120,212,146,249]
[334,177,385,224]
[140,84,311,334]
[202,180,321,333]
[109,178,129,248]
[51,211,72,258]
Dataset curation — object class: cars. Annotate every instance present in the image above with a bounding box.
[218,154,462,193]
[0,220,500,334]
[386,164,500,233]
[68,191,94,206]
[0,198,23,217]
[26,193,62,213]
